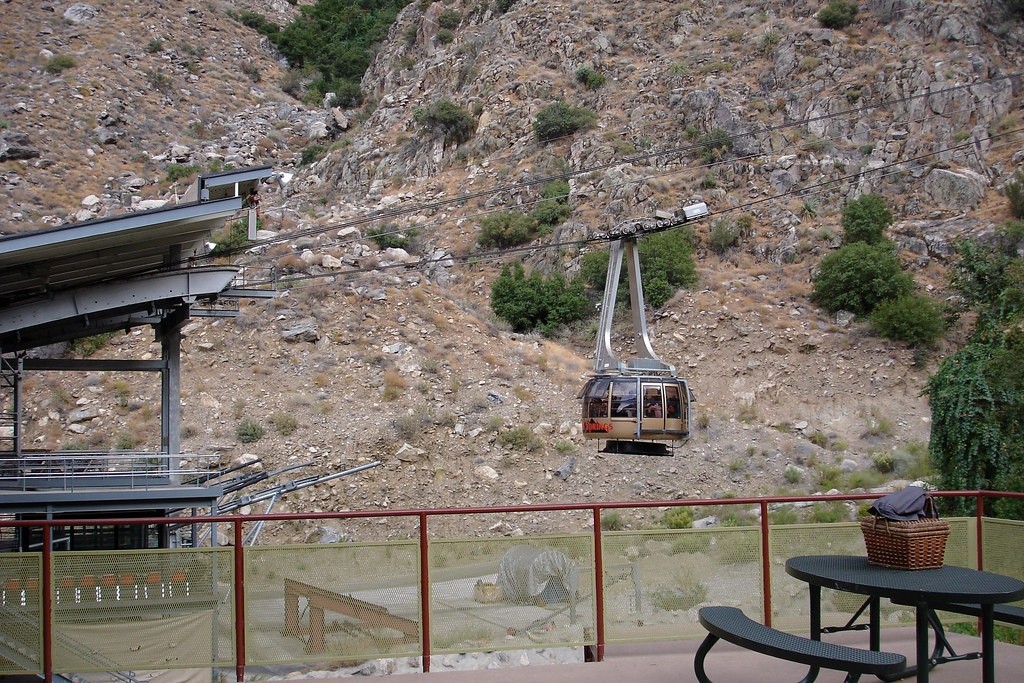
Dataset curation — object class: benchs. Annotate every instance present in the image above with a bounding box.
[694,607,906,683]
[889,598,1024,671]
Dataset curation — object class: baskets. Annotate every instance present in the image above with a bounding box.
[861,495,952,572]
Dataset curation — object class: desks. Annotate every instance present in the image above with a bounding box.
[785,555,1024,683]
